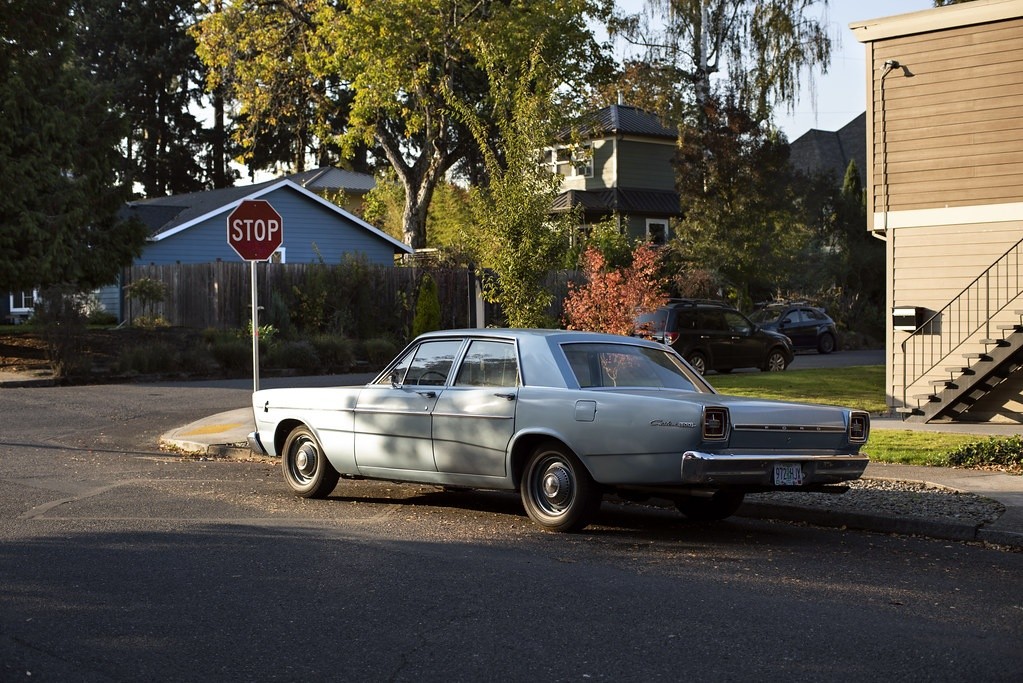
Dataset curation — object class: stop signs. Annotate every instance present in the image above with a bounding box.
[226,200,284,260]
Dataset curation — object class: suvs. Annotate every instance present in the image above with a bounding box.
[627,299,797,376]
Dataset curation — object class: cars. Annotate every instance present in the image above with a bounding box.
[246,327,872,536]
[740,304,840,358]
